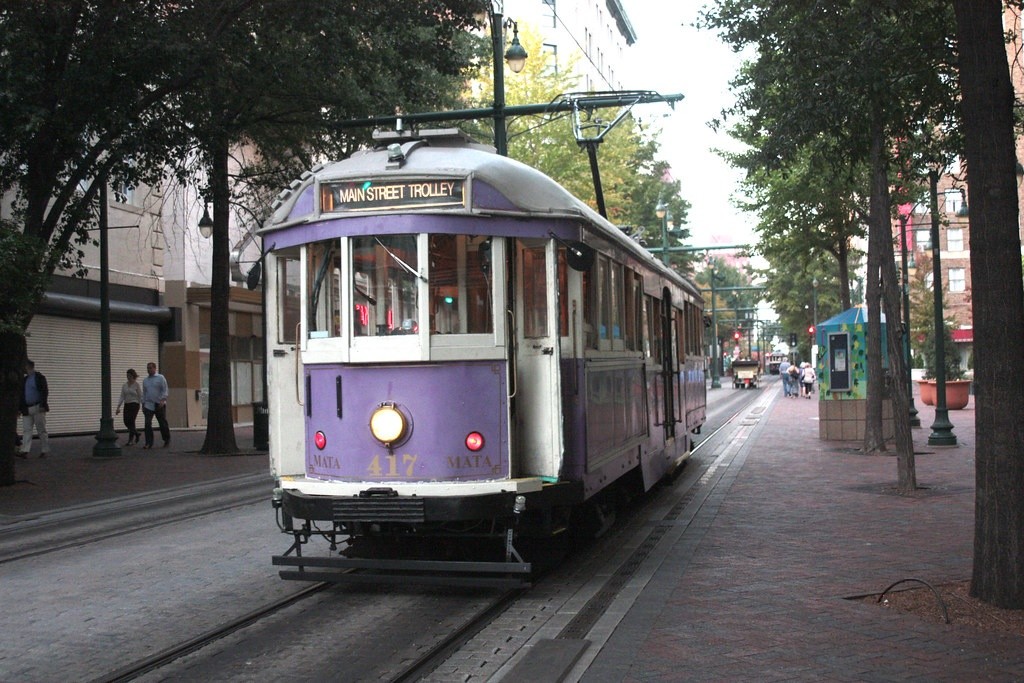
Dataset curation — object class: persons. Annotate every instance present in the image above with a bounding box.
[736,351,745,362]
[778,357,816,399]
[141,362,171,448]
[116,369,143,445]
[15,359,50,459]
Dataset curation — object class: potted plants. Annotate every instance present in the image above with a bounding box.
[904,245,973,410]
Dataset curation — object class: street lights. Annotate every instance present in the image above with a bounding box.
[900,188,969,423]
[812,279,818,324]
[197,196,269,409]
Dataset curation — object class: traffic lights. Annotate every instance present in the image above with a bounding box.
[734,332,740,339]
[790,333,797,346]
[808,326,815,334]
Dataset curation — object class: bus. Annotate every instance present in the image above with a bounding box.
[769,351,787,375]
[247,88,712,591]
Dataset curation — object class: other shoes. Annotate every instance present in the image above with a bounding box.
[15,451,28,459]
[135,433,141,443]
[143,442,154,449]
[39,452,51,458]
[125,442,134,446]
[163,439,170,448]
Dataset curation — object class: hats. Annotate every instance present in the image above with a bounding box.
[800,361,806,369]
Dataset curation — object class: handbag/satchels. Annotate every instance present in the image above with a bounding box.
[801,369,806,381]
[790,366,800,379]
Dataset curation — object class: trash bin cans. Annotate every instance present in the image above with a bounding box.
[251,400,269,452]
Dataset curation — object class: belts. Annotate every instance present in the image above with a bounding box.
[26,402,41,408]
